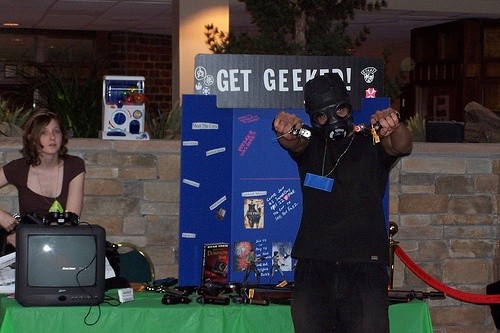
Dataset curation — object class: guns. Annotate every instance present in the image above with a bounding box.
[354,113,405,138]
[272,117,313,140]
[236,281,445,306]
[146,283,199,306]
[196,286,234,306]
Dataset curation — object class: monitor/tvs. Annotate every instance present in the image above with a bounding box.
[15,224,106,307]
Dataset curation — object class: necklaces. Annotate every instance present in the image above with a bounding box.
[35,160,63,213]
[303,133,355,192]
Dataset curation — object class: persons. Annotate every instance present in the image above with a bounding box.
[274,73,412,333]
[0,111,86,254]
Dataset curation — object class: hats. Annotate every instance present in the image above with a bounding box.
[303,72,350,115]
